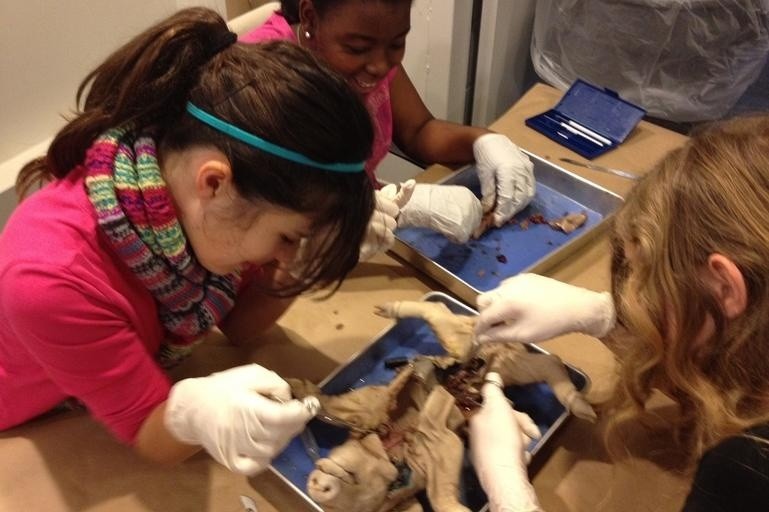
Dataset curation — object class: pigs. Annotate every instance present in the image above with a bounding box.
[279,300,598,512]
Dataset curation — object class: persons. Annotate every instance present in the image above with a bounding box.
[467,109,767,512]
[0,8,416,480]
[236,0,537,245]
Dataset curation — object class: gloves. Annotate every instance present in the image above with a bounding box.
[473,133,536,229]
[164,363,311,478]
[286,179,415,290]
[471,273,617,346]
[467,382,545,512]
[398,181,483,244]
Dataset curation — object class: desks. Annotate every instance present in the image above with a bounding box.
[0,81,736,510]
